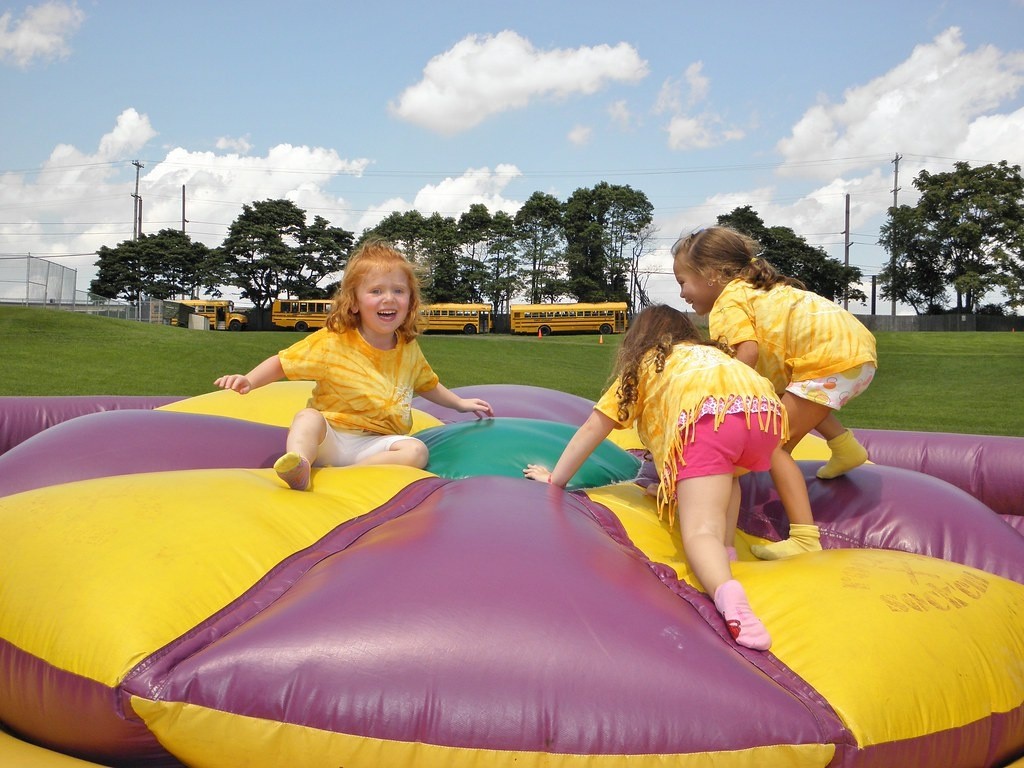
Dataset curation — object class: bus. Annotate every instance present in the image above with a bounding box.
[170,301,249,332]
[510,303,630,334]
[272,298,339,331]
[414,301,494,335]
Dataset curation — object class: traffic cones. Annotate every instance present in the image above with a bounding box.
[538,329,542,339]
[599,335,604,344]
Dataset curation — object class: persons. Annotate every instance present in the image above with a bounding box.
[214,239,496,491]
[671,226,877,561]
[523,303,791,650]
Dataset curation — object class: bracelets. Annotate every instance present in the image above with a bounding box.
[547,474,568,489]
